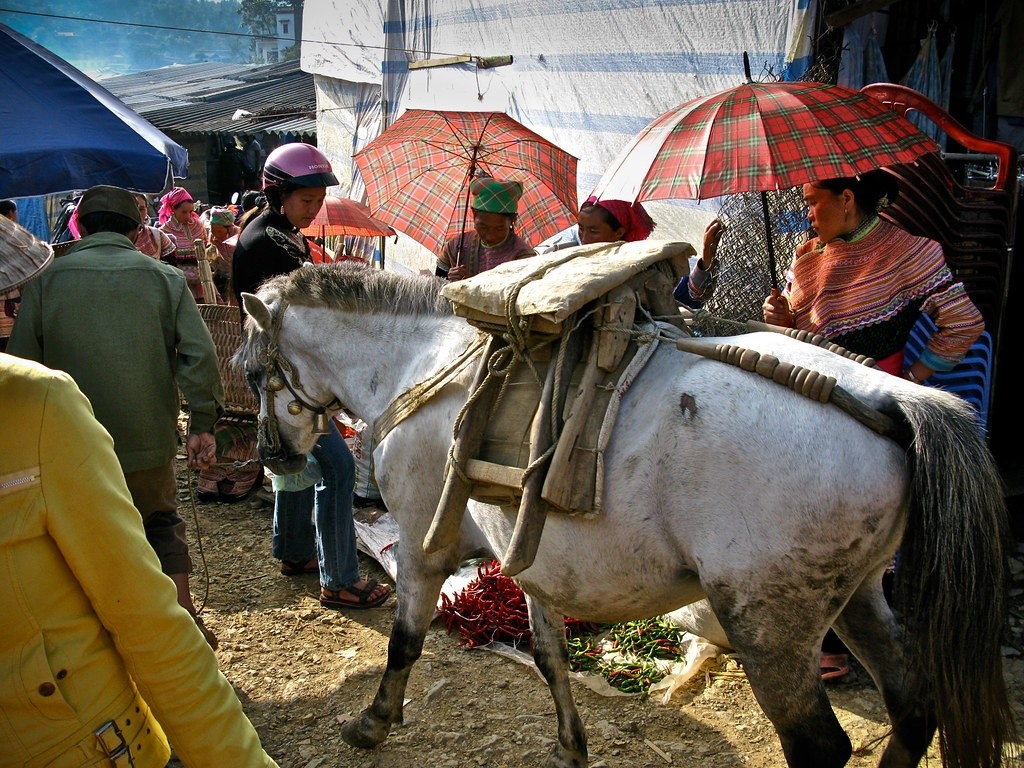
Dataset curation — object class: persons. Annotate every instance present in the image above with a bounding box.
[0,199,17,223]
[0,215,284,768]
[761,169,985,681]
[129,187,268,306]
[578,186,727,310]
[7,185,227,650]
[230,143,391,609]
[436,177,536,283]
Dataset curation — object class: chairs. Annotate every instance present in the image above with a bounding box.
[853,83,1019,449]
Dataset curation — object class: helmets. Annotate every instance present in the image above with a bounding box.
[264,141,339,203]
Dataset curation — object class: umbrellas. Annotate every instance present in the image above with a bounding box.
[584,50,942,305]
[0,23,190,204]
[351,107,580,279]
[299,195,399,266]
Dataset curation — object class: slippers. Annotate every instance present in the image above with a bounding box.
[814,653,850,680]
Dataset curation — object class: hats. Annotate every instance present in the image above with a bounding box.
[0,213,56,297]
[470,177,522,212]
[211,209,235,226]
[77,184,145,228]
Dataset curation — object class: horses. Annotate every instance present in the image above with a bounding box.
[224,225,1018,768]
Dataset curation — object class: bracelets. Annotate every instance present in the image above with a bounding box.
[905,368,921,385]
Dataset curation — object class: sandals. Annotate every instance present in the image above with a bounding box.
[280,553,320,577]
[319,578,392,609]
